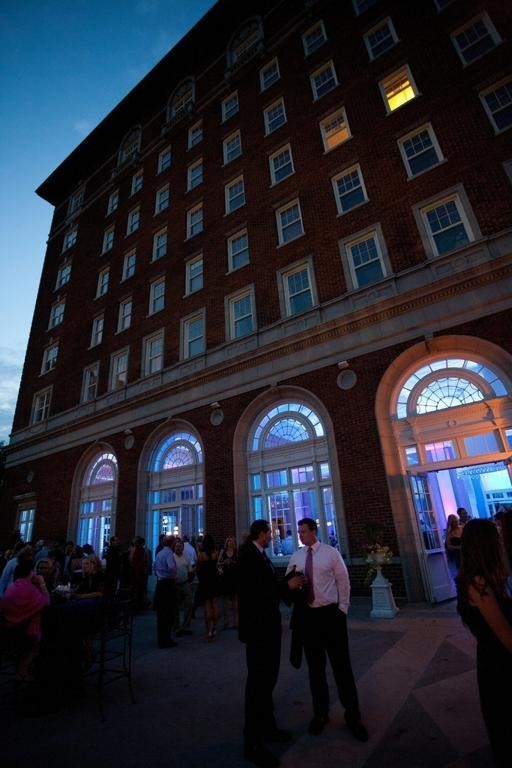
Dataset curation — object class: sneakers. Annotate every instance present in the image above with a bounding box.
[345,720,369,741]
[307,714,329,735]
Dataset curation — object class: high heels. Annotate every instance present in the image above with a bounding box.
[200,630,217,642]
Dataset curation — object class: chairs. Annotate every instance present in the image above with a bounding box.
[2,584,140,723]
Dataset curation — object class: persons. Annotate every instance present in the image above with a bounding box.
[443,507,512,766]
[236,519,309,768]
[282,518,369,741]
[0,530,236,683]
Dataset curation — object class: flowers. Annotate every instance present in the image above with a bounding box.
[351,516,393,581]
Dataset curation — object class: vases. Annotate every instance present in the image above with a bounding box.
[365,549,389,582]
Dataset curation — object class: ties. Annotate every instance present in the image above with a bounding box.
[304,547,314,605]
